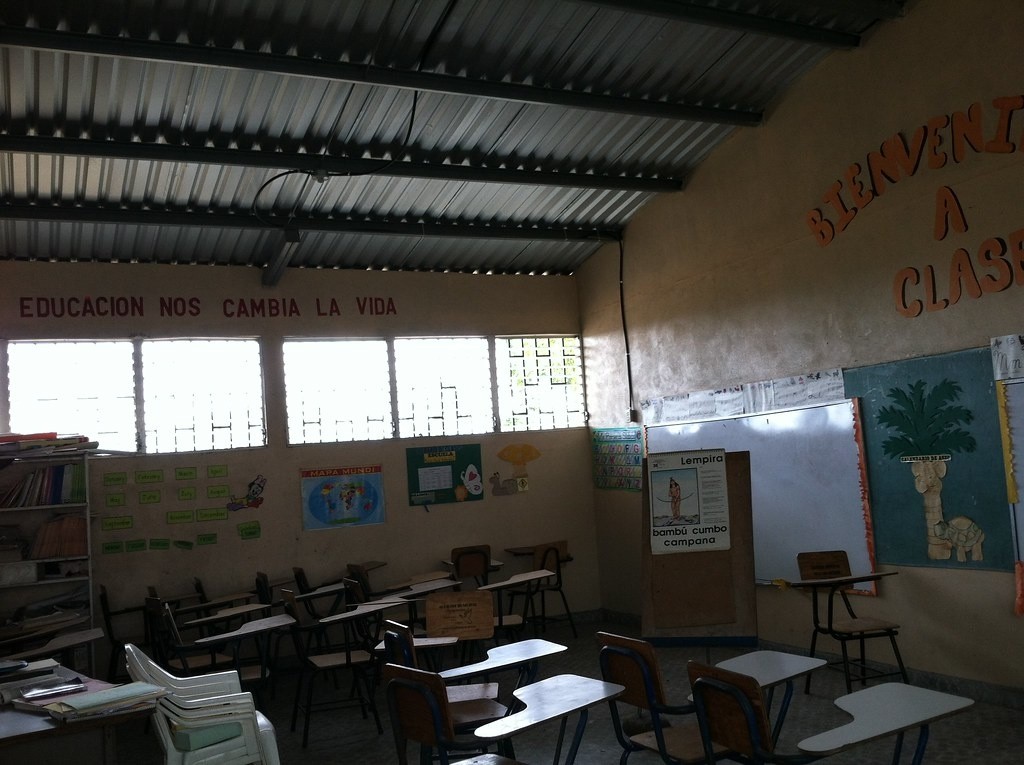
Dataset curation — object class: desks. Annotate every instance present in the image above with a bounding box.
[791,571,899,630]
[688,650,828,754]
[319,597,414,646]
[504,546,574,586]
[441,557,508,587]
[0,658,165,765]
[194,614,292,686]
[382,579,463,600]
[176,593,258,619]
[797,682,977,765]
[476,569,551,647]
[474,674,631,764]
[437,639,572,717]
[294,582,346,617]
[393,571,451,589]
[182,604,269,631]
[374,636,456,651]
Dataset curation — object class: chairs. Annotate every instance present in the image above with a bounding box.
[99,527,578,749]
[385,663,527,765]
[380,619,518,761]
[686,660,774,765]
[124,643,281,764]
[595,630,795,765]
[791,550,912,694]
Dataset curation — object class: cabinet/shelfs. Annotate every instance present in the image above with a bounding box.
[0,450,105,680]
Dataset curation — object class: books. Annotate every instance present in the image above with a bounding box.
[0,657,174,722]
[0,462,87,508]
[0,429,101,462]
[0,510,87,564]
[0,584,90,642]
